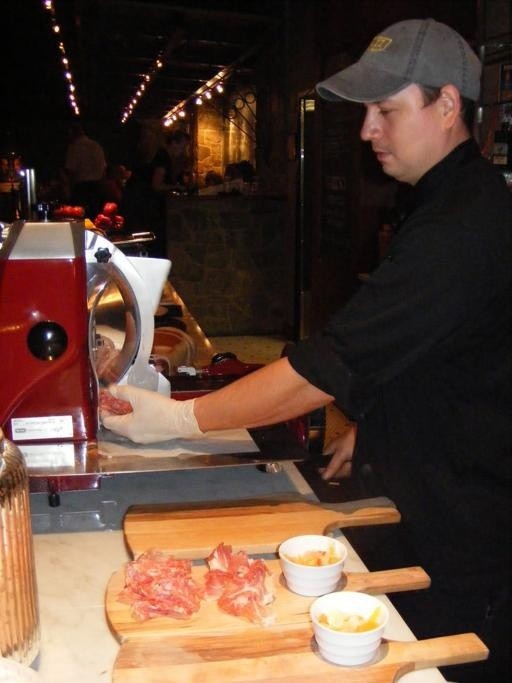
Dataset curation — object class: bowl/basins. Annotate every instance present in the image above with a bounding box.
[1,181,21,191]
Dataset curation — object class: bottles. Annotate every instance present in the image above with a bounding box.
[0,426,41,665]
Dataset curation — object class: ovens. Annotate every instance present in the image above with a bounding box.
[2,215,311,509]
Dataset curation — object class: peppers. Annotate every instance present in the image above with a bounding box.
[53,205,84,216]
[95,202,125,230]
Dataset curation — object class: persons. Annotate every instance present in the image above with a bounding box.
[98,16,510,682]
[2,121,257,260]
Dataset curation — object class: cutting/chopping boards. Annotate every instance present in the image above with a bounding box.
[105,561,433,643]
[123,496,402,561]
[110,633,490,681]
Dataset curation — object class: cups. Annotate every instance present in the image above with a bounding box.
[123,256,172,315]
[310,591,390,665]
[278,534,349,598]
[19,167,38,219]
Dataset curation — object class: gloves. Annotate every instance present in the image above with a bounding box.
[99,382,209,445]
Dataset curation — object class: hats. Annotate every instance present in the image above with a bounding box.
[314,19,486,105]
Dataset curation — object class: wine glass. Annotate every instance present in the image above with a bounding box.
[3,150,20,180]
[38,202,52,220]
